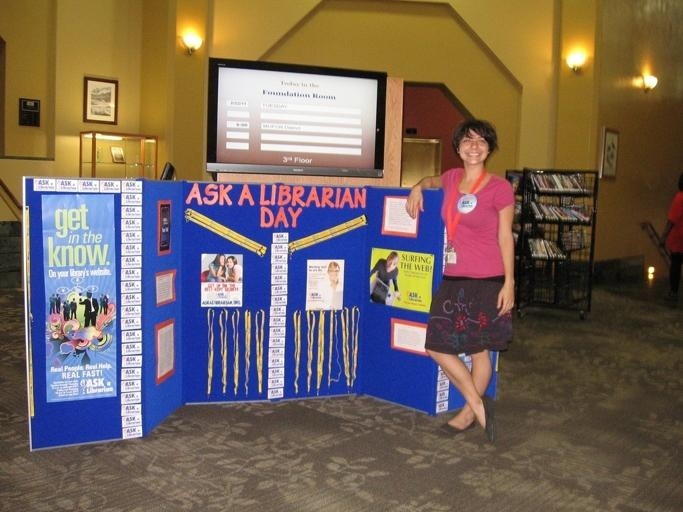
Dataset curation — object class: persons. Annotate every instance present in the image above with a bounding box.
[207,255,226,282]
[370,251,400,306]
[658,174,683,304]
[318,262,344,310]
[223,256,243,283]
[49,293,108,327]
[405,121,516,446]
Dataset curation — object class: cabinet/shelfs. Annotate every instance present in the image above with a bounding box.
[505,168,599,321]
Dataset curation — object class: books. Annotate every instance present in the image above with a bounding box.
[516,175,594,262]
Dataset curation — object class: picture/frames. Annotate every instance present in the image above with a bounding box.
[110,146,126,163]
[598,126,620,180]
[83,76,118,125]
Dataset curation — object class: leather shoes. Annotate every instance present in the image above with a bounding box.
[481,396,497,445]
[433,419,480,438]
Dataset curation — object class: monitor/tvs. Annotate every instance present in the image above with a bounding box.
[207,57,387,178]
[160,162,175,180]
